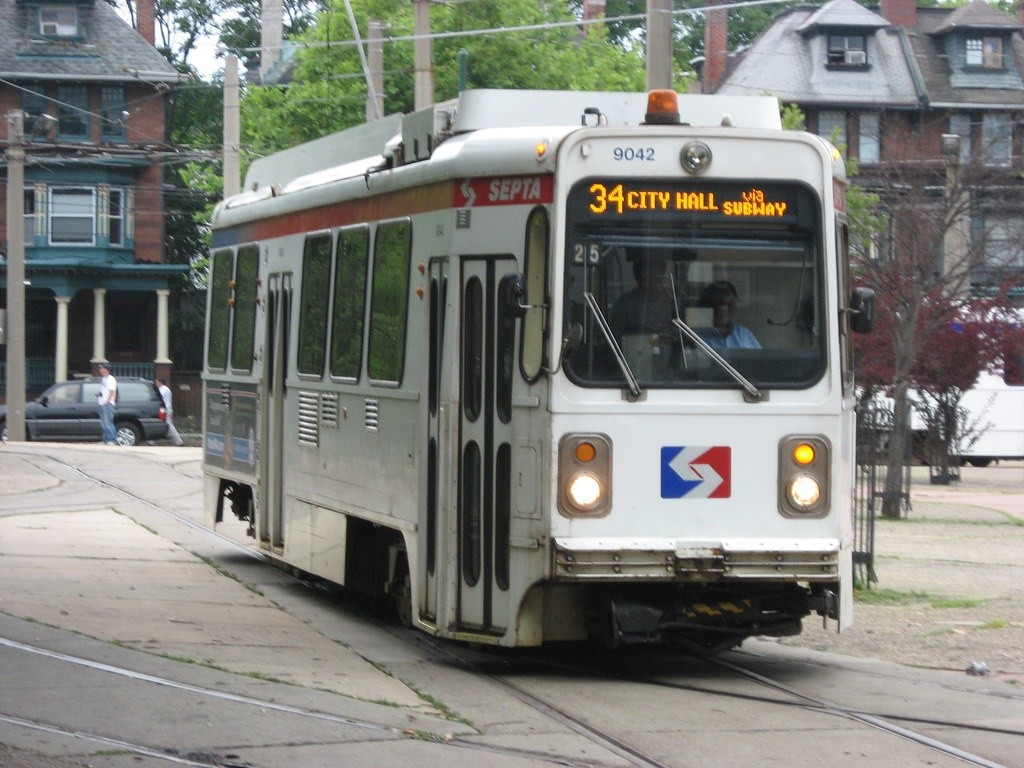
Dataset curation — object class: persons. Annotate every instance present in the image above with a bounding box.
[145,376,184,447]
[685,281,762,349]
[98,363,118,445]
[611,255,688,377]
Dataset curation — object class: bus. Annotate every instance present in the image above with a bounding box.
[200,84,877,662]
[855,306,1023,462]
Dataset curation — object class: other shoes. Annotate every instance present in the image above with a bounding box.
[105,441,115,445]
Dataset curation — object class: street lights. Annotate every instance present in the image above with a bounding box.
[5,113,60,439]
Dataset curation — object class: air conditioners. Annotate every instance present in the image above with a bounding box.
[844,51,866,64]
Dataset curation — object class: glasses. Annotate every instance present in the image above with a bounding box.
[716,298,740,308]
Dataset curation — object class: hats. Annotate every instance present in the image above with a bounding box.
[98,364,112,371]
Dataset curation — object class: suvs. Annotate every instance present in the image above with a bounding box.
[0,376,169,447]
